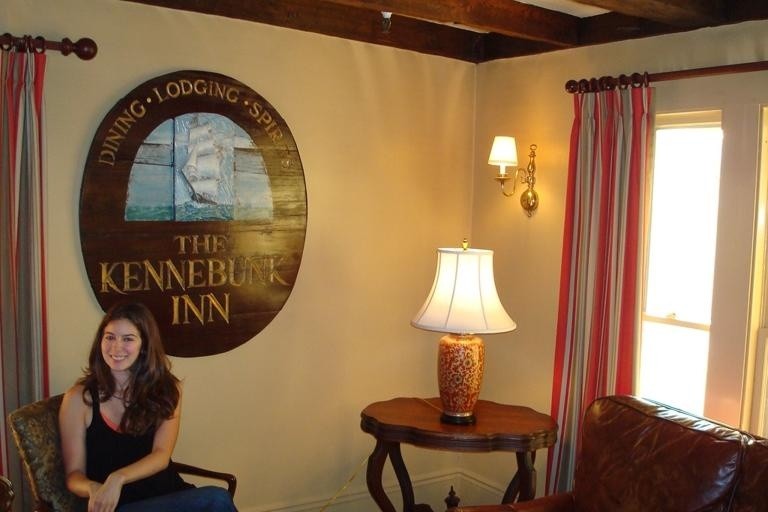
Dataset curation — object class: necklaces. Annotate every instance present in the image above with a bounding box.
[112,373,133,398]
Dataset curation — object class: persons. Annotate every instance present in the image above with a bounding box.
[57,298,238,512]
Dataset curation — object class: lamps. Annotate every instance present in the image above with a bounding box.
[409,235,518,425]
[487,133,540,219]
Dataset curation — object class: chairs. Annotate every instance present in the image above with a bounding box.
[450,393,768,511]
[0,475,16,512]
[6,392,237,512]
